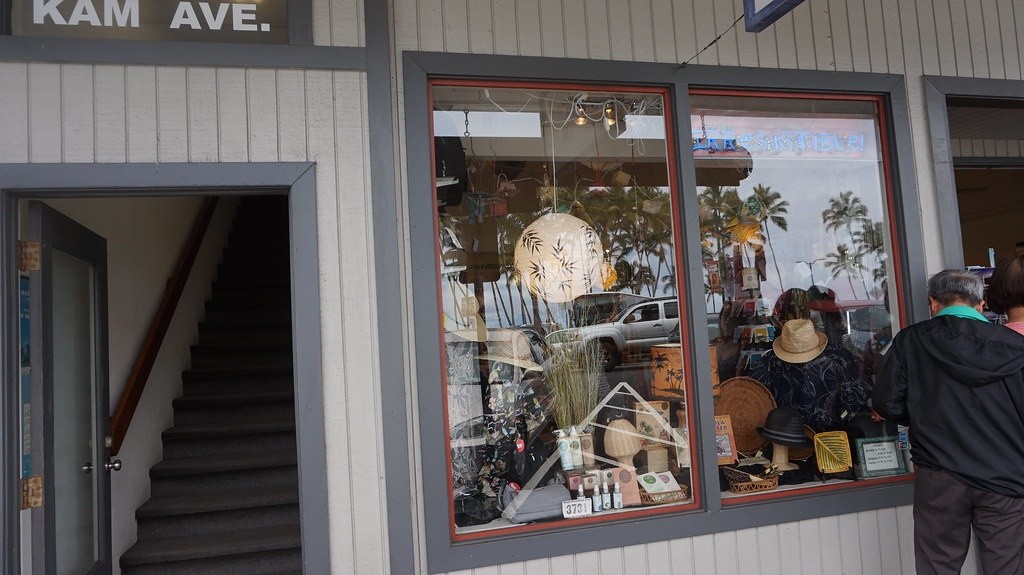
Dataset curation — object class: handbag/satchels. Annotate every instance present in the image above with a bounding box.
[498,476,572,524]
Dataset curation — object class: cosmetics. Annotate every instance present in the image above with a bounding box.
[577,483,586,501]
[612,481,626,509]
[600,481,613,510]
[565,423,584,470]
[552,427,576,471]
[591,484,605,511]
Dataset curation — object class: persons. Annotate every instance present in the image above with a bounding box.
[867,267,1024,574]
[983,248,1024,336]
[710,267,898,481]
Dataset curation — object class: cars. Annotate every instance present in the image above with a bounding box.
[442,293,721,479]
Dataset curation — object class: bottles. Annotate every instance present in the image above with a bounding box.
[552,426,622,512]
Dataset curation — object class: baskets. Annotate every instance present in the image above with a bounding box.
[712,376,778,457]
[729,474,778,494]
[787,424,817,461]
[638,484,690,506]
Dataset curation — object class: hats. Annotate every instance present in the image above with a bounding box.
[806,285,843,314]
[771,319,829,364]
[756,404,814,448]
